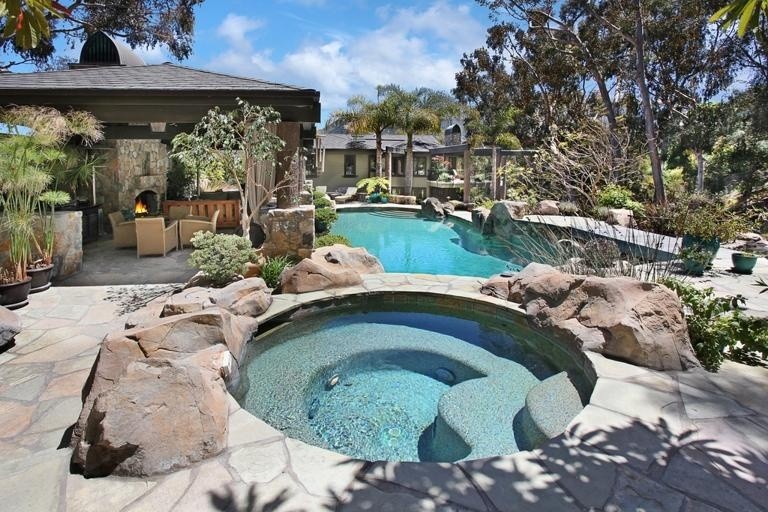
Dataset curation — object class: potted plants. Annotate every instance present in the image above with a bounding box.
[160,96,309,279]
[667,189,757,275]
[0,102,106,311]
[355,176,390,204]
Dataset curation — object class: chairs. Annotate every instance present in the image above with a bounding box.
[108,206,221,260]
[315,185,358,206]
